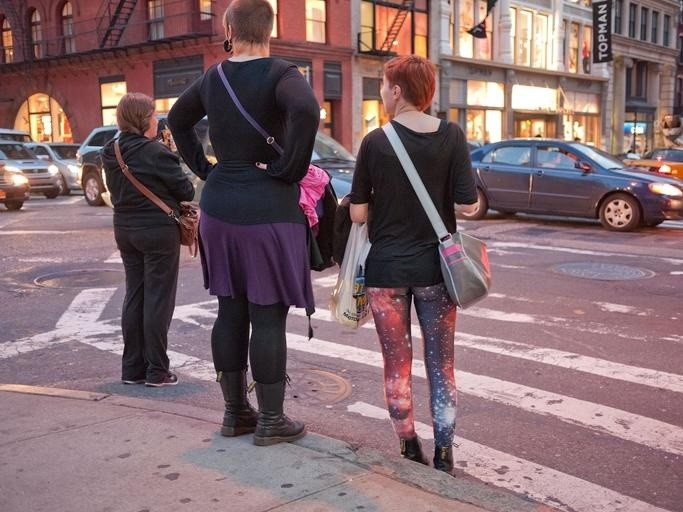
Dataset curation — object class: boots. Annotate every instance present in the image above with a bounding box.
[433,446,455,476]
[254,379,306,446]
[400,437,430,465]
[216,365,258,436]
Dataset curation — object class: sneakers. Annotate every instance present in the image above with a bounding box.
[144,374,179,387]
[122,377,146,384]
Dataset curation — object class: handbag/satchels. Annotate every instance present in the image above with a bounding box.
[177,202,201,245]
[314,194,325,219]
[438,230,491,311]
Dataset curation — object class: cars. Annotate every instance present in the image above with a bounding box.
[0,111,81,211]
[453,133,682,233]
[309,131,354,205]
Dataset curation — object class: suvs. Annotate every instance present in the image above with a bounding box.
[74,124,119,208]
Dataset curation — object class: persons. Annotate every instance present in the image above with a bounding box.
[348,54,480,472]
[166,0,319,446]
[98,92,195,388]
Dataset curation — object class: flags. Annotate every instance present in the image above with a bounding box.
[467,1,497,39]
[591,0,613,64]
